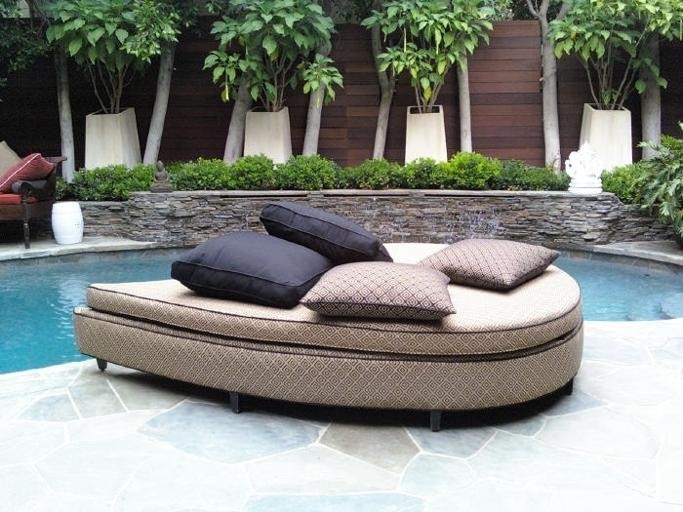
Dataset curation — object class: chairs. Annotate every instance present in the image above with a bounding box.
[0,155,68,250]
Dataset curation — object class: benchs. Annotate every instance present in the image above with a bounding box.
[70,241,585,434]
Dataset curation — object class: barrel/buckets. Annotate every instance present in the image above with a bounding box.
[51,200,84,244]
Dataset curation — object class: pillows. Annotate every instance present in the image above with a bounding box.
[0,139,56,195]
